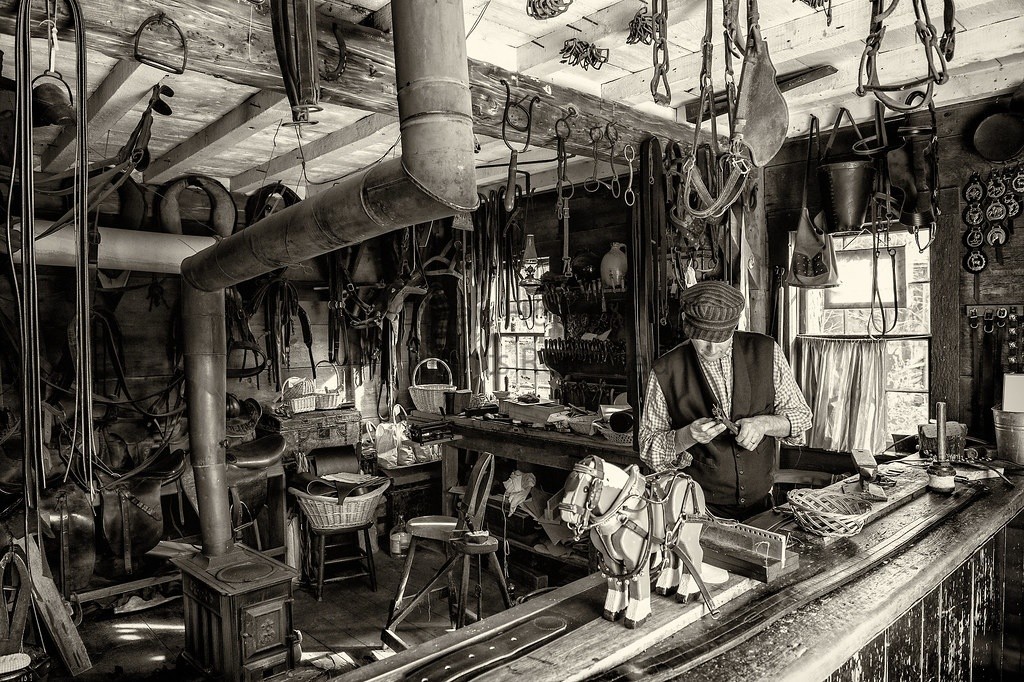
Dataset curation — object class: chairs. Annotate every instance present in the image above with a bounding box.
[380,452,495,650]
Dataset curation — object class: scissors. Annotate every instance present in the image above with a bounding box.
[712,401,741,436]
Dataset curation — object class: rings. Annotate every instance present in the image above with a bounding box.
[750,441,755,445]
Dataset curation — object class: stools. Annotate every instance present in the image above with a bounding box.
[310,521,378,602]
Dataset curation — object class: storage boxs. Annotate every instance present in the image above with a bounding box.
[275,408,359,452]
[485,505,538,537]
[508,404,564,424]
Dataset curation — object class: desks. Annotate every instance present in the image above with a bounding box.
[406,410,639,575]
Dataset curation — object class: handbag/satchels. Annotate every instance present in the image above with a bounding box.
[786,206,842,288]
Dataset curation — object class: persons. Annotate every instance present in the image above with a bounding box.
[638,280,815,522]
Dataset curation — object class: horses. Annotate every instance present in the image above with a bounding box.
[558,454,722,630]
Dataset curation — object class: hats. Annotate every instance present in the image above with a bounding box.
[680,281,745,342]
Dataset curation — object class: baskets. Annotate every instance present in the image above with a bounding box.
[313,360,343,409]
[280,376,315,414]
[569,416,599,435]
[408,358,457,415]
[288,467,391,530]
[597,425,633,446]
[786,488,873,537]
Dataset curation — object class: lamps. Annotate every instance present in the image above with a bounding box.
[519,234,543,295]
